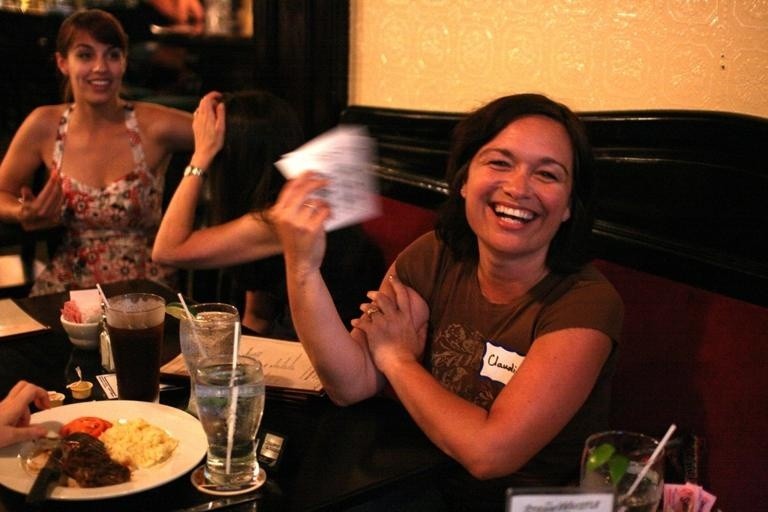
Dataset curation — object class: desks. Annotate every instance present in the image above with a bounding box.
[0,277,452,511]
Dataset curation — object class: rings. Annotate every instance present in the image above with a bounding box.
[53,216,60,223]
[303,203,317,211]
[366,305,379,319]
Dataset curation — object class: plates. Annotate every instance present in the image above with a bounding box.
[0,396,209,500]
[191,465,269,496]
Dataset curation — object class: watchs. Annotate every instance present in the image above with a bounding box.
[182,164,208,180]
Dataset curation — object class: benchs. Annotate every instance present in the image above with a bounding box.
[357,161,768,511]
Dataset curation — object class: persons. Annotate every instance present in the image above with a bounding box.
[265,91,627,511]
[151,90,387,342]
[0,8,194,298]
[1,379,53,447]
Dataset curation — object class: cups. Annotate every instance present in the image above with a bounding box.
[580,430,666,511]
[103,292,164,404]
[191,356,264,484]
[177,304,243,412]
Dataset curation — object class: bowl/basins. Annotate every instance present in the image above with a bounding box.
[59,311,101,350]
